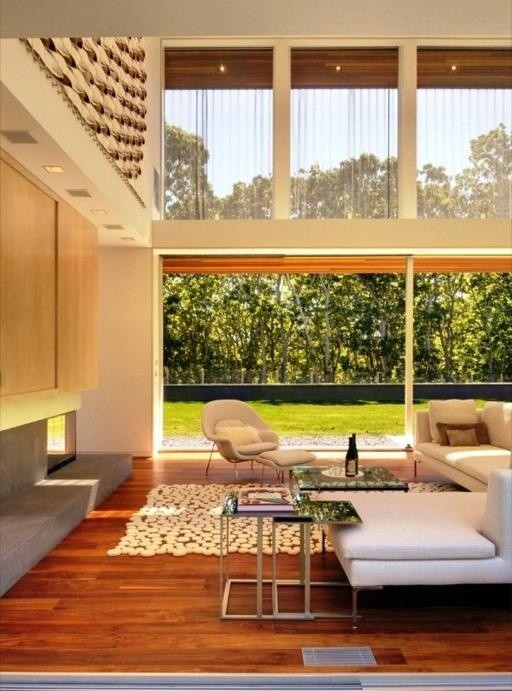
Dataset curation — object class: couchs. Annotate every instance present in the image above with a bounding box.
[316,470,512,629]
[413,402,512,492]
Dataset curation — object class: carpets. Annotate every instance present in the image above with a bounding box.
[107,483,460,558]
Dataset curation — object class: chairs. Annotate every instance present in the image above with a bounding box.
[200,400,278,481]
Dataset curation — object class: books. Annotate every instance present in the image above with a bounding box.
[235,487,295,513]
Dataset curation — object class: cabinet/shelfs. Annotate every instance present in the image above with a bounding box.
[220,504,364,621]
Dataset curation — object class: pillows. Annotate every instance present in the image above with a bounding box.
[214,427,262,447]
[428,398,476,447]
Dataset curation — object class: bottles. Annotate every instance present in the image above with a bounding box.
[343,433,358,478]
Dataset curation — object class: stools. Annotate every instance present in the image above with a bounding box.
[255,449,317,484]
